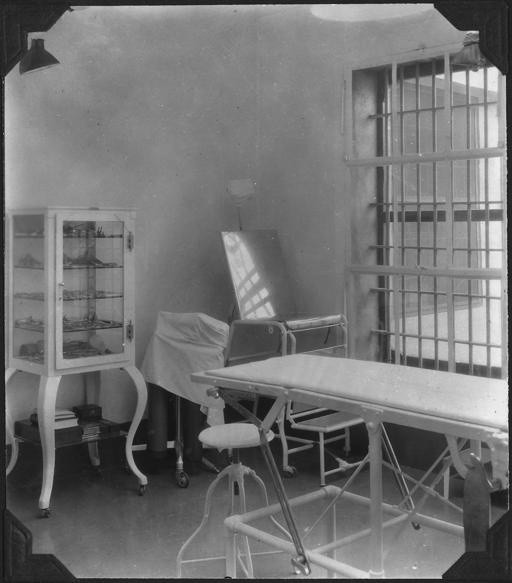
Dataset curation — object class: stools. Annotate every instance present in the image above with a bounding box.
[176,423,299,579]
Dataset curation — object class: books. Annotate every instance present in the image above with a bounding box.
[29,409,79,430]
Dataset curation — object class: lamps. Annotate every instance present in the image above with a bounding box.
[19,39,61,77]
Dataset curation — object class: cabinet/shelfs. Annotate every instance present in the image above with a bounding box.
[5,209,149,517]
[155,309,370,487]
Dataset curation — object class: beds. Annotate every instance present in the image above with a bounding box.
[188,353,511,578]
